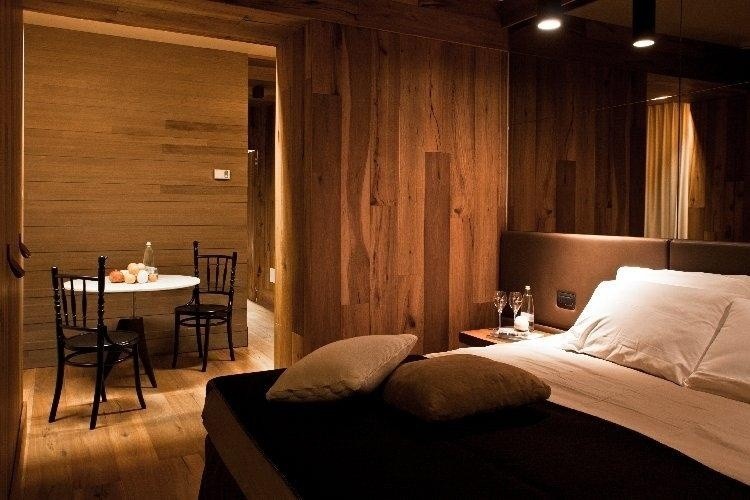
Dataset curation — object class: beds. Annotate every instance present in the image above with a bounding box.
[201,231,750,498]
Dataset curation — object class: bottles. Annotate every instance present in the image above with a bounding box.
[523,285,535,332]
[143,240,154,269]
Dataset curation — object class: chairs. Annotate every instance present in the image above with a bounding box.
[172,241,237,373]
[48,256,157,430]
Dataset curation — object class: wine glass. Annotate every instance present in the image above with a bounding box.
[509,291,523,326]
[492,290,507,334]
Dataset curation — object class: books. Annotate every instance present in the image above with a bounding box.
[496,328,551,343]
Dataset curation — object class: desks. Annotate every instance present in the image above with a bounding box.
[459,326,551,347]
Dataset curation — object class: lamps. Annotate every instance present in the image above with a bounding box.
[631,1,656,49]
[536,0,562,32]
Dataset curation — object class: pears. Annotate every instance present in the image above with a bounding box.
[121,263,158,284]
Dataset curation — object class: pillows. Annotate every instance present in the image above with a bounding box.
[554,265,750,405]
[266,334,552,424]
